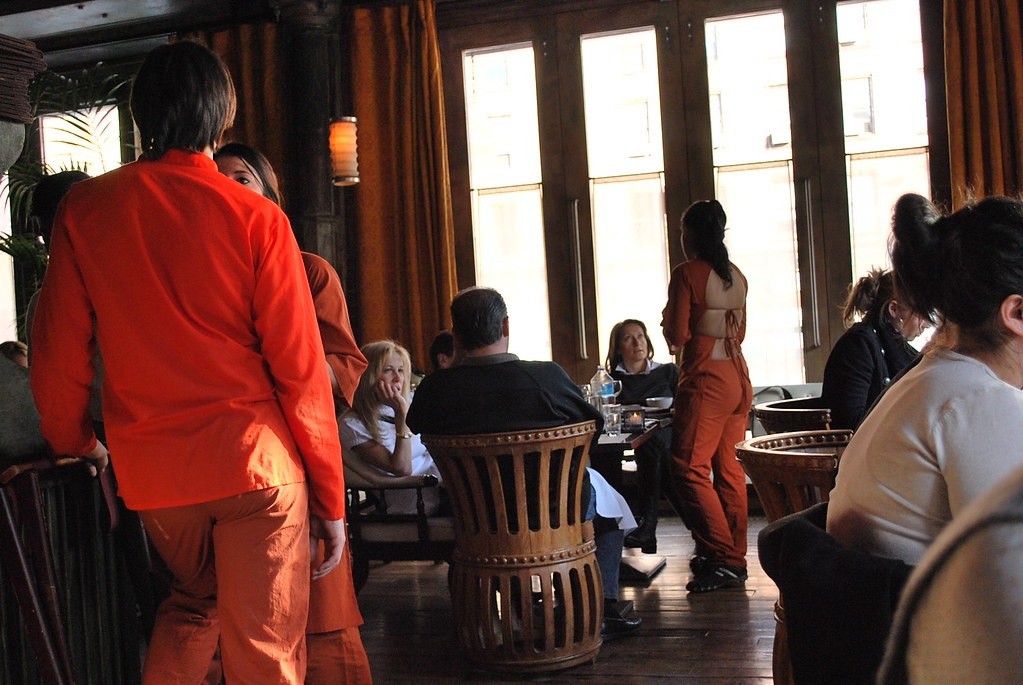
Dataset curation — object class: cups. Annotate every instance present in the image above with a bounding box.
[604,403,621,437]
[586,397,603,412]
[575,384,590,402]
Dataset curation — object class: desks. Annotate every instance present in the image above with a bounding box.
[590,419,667,578]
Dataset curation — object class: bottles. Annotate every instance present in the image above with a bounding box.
[590,365,622,404]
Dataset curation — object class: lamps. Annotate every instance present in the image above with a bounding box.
[325,113,361,189]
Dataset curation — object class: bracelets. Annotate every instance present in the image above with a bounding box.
[396,431,413,439]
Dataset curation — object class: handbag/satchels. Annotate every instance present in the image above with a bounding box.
[749,385,793,437]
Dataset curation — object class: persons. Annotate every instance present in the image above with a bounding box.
[658,201,754,591]
[209,140,377,685]
[868,458,1022,685]
[823,191,1023,569]
[30,35,347,685]
[336,339,546,623]
[426,331,455,369]
[20,171,110,450]
[401,286,643,639]
[601,317,682,555]
[820,266,932,436]
[0,341,29,368]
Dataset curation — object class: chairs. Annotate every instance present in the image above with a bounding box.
[735,430,854,685]
[754,397,831,434]
[1,458,156,685]
[420,418,604,675]
[340,446,454,594]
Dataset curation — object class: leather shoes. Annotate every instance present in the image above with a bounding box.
[588,616,643,641]
[515,590,544,620]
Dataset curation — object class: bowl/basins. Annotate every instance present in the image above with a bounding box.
[646,396,673,409]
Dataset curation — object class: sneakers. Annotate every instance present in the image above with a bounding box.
[685,564,749,593]
[691,554,745,587]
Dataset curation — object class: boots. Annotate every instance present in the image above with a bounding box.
[624,516,659,554]
